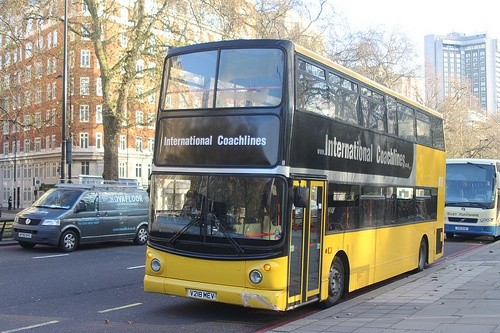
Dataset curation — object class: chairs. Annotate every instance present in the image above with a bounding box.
[328,197,430,232]
[448,182,491,201]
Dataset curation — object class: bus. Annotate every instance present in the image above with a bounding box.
[143,40,445,313]
[445,159,500,243]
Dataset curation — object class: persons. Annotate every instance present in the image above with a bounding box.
[8,194,12,210]
[61,193,72,207]
[328,194,437,231]
[180,190,202,219]
[0,204,2,217]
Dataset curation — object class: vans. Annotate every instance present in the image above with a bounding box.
[12,186,152,253]
